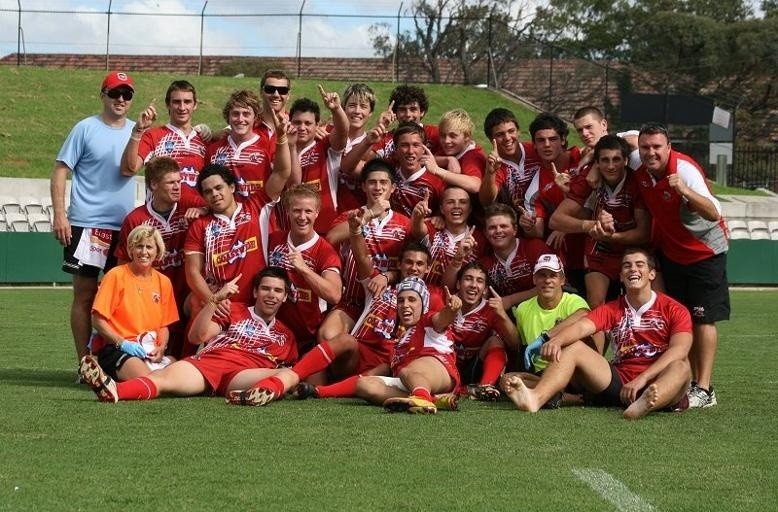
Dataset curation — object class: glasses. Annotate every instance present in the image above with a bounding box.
[262,85,290,95]
[103,89,133,101]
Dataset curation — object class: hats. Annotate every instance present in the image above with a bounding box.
[101,72,135,93]
[396,276,431,314]
[532,253,565,275]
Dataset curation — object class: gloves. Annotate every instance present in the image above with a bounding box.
[120,340,146,359]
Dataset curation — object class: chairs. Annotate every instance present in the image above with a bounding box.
[722,218,778,241]
[0,194,145,235]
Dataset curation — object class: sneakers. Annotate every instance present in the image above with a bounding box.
[293,382,319,399]
[660,381,717,412]
[383,397,438,414]
[77,354,118,404]
[226,387,274,407]
[467,382,500,402]
[539,389,565,410]
[434,394,459,410]
